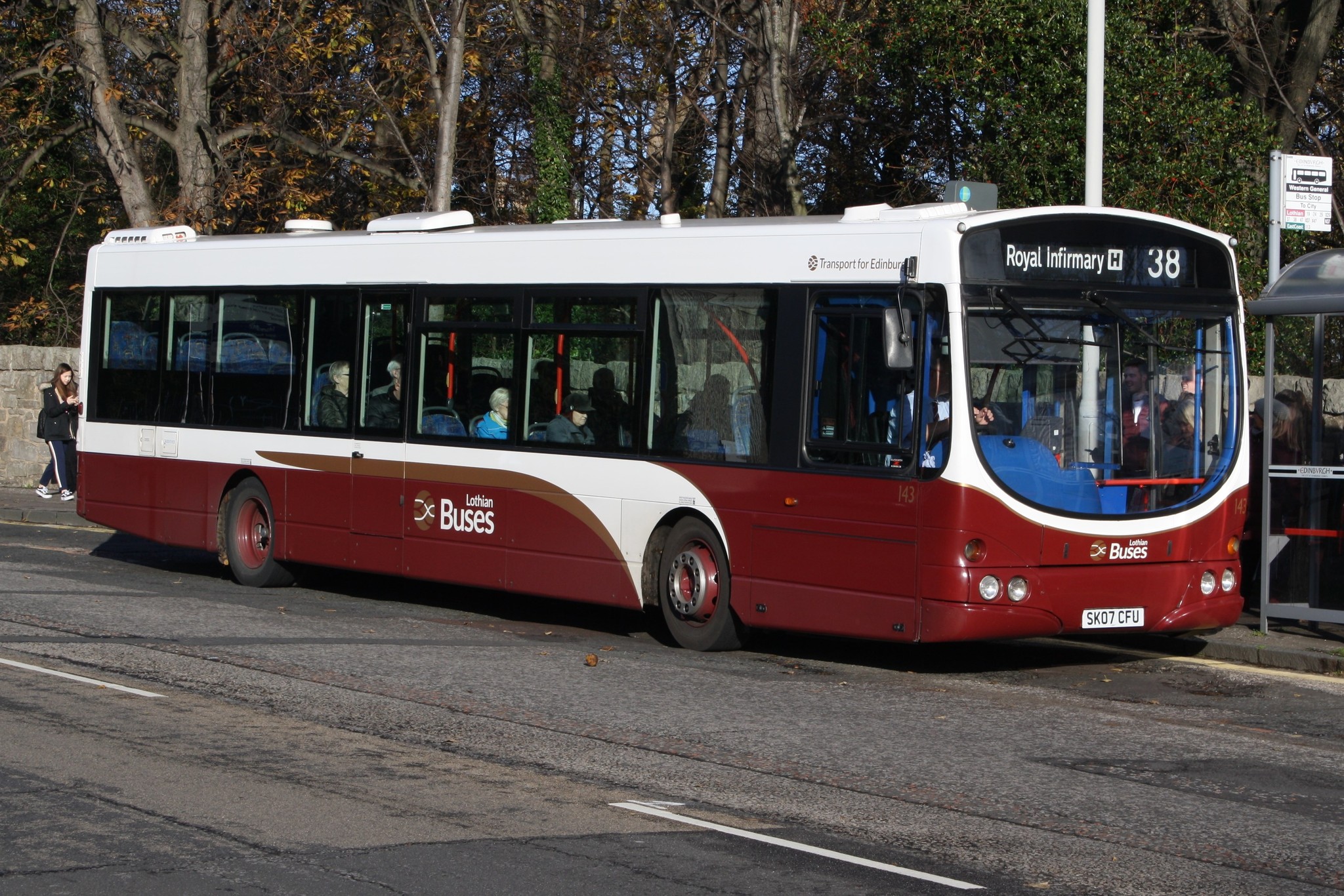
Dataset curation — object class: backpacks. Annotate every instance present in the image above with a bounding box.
[37,407,47,439]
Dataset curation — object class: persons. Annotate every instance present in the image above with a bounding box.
[36,363,80,501]
[318,356,738,462]
[1112,361,1344,616]
[884,356,993,472]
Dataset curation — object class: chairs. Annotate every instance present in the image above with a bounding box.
[103,319,768,460]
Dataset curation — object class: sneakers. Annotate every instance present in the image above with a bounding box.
[35,485,52,499]
[60,489,75,501]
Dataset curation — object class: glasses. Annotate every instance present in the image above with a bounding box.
[498,403,513,412]
[1176,419,1189,426]
[1182,376,1194,382]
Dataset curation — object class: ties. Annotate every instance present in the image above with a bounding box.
[933,399,939,423]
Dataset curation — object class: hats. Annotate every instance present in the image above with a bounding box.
[562,393,596,413]
[1255,397,1289,425]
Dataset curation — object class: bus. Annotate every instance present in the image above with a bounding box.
[74,202,1251,653]
